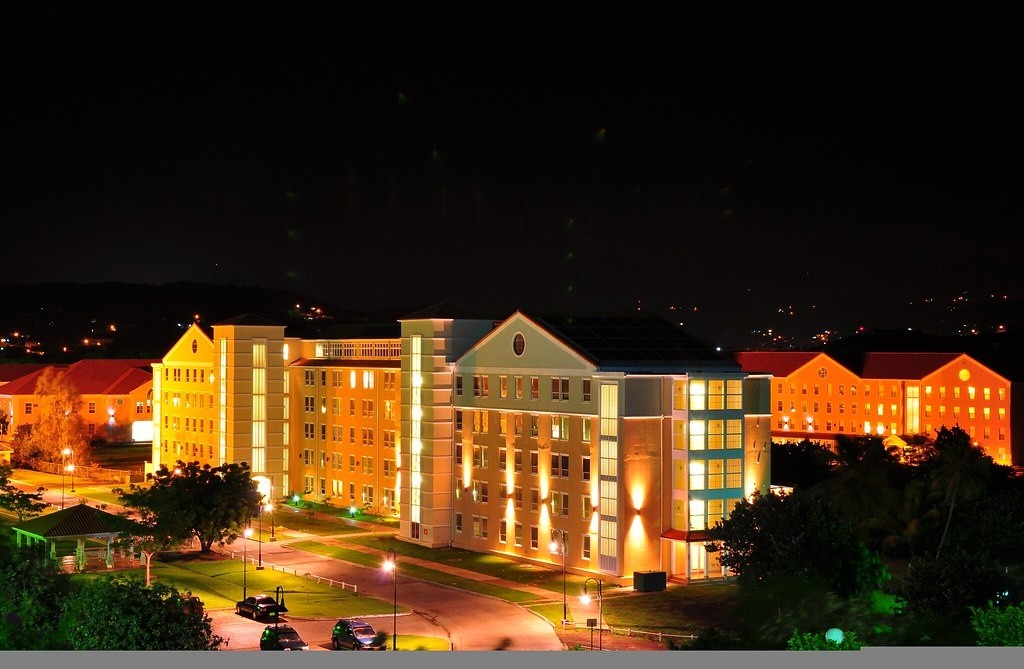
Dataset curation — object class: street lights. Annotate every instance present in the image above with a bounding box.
[242,528,253,600]
[578,577,603,652]
[261,474,276,541]
[63,448,75,491]
[383,548,398,651]
[273,585,289,650]
[548,528,567,625]
[62,463,75,510]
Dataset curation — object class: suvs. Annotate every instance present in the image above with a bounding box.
[259,624,310,651]
[330,618,387,651]
[235,594,279,620]
